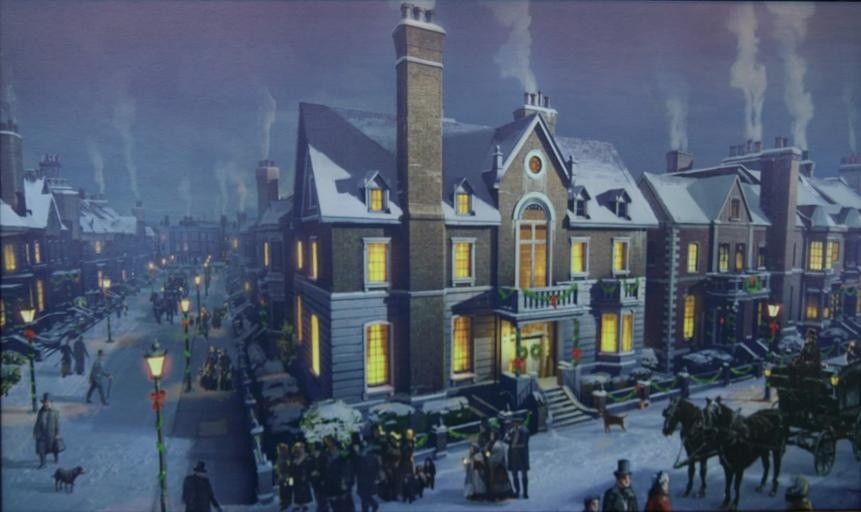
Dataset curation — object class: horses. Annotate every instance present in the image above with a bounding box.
[703,396,788,512]
[662,394,721,498]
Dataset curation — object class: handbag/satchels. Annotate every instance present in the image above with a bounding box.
[54,439,66,453]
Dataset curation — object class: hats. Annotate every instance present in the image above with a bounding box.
[39,393,53,403]
[192,462,206,472]
[510,414,523,421]
[805,328,817,340]
[614,460,632,475]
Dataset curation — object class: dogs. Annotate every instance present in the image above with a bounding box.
[596,406,629,433]
[51,465,86,493]
